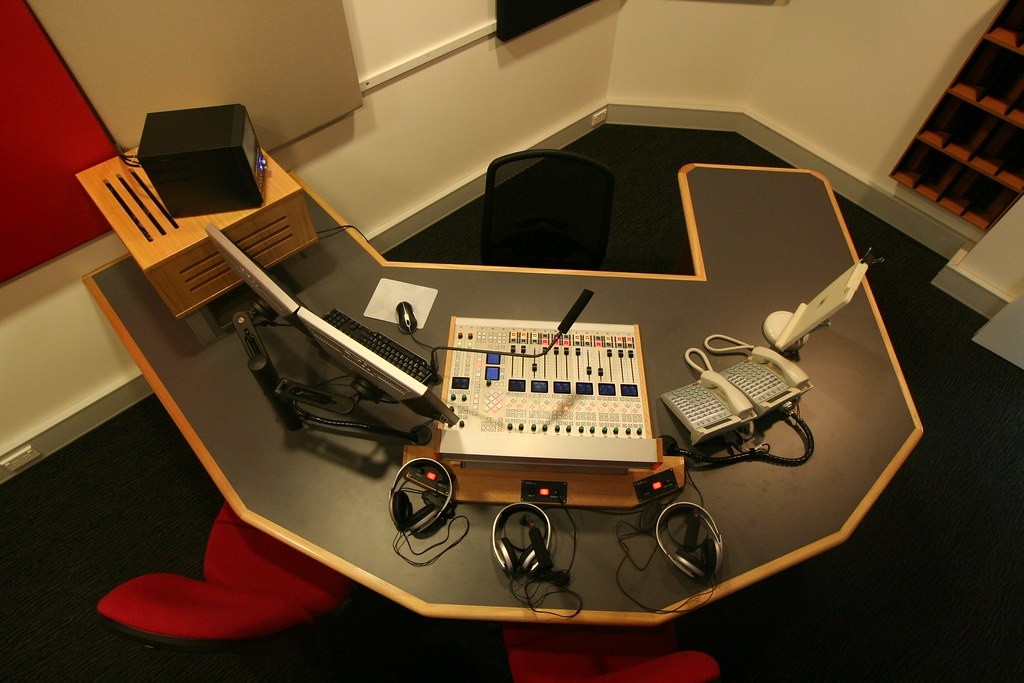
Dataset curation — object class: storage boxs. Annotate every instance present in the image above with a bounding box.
[76,146,319,319]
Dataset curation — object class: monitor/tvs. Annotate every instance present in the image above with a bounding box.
[207,225,461,427]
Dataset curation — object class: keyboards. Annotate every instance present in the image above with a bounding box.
[322,308,432,385]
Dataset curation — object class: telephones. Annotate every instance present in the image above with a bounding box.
[718,345,815,420]
[660,370,757,447]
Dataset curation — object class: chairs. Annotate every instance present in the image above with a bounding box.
[500,619,721,683]
[95,497,355,652]
[480,150,615,272]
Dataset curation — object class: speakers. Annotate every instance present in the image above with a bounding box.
[137,103,266,219]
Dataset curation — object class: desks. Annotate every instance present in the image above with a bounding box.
[82,163,923,627]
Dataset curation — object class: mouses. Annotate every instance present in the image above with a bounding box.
[396,302,417,333]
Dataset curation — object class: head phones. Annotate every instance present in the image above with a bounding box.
[492,502,552,575]
[388,458,453,533]
[656,501,723,578]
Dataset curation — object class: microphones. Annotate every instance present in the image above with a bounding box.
[683,513,700,553]
[431,288,595,386]
[524,514,553,572]
